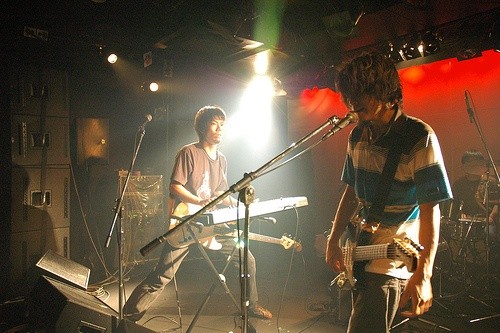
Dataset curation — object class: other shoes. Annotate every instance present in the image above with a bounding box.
[250,302,272,319]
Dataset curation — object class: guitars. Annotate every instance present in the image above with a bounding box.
[333,221,425,287]
[167,200,303,252]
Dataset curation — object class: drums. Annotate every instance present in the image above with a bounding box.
[455,219,486,241]
[437,197,464,224]
[431,235,452,277]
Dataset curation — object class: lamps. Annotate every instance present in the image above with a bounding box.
[385,31,448,63]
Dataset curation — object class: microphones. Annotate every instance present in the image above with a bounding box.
[136,114,152,132]
[465,91,474,124]
[322,111,360,141]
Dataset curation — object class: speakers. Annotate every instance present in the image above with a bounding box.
[0,59,166,333]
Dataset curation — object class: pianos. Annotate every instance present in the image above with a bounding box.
[178,195,309,333]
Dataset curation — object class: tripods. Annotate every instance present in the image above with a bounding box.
[431,117,500,311]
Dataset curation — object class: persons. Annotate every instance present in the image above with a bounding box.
[122,105,272,323]
[326,51,454,333]
[442,149,500,262]
